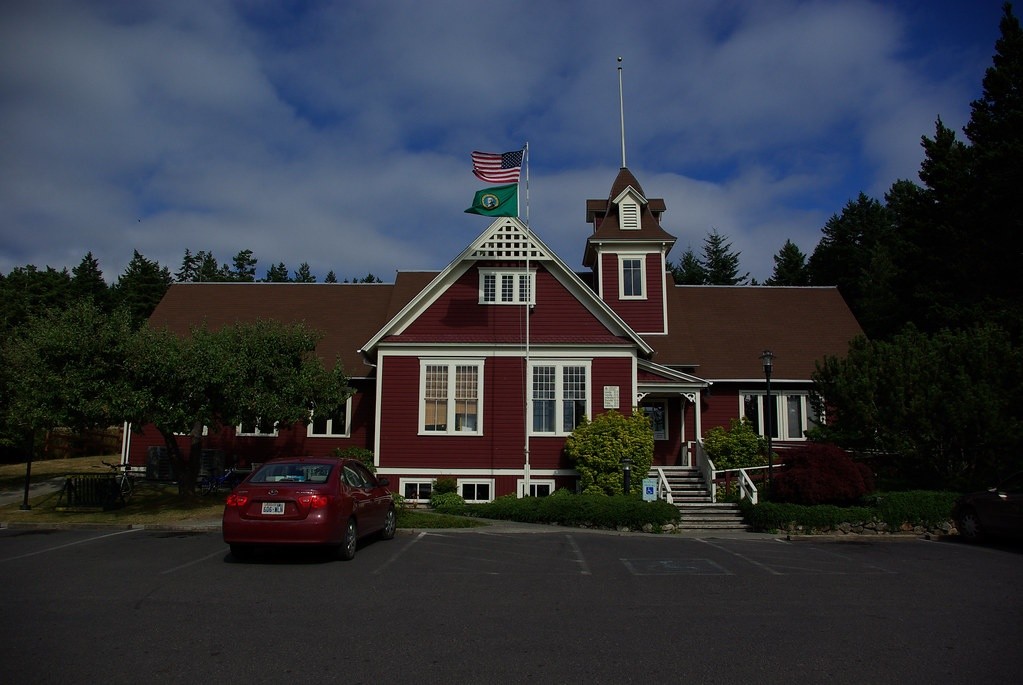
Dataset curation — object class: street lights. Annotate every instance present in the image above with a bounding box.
[758,349,777,501]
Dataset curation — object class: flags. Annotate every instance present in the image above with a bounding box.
[471,150,524,183]
[464,183,519,217]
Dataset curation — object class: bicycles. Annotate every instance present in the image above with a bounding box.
[192,463,239,498]
[102,460,136,511]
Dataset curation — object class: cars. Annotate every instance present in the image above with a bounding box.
[951,470,1023,540]
[223,456,396,560]
